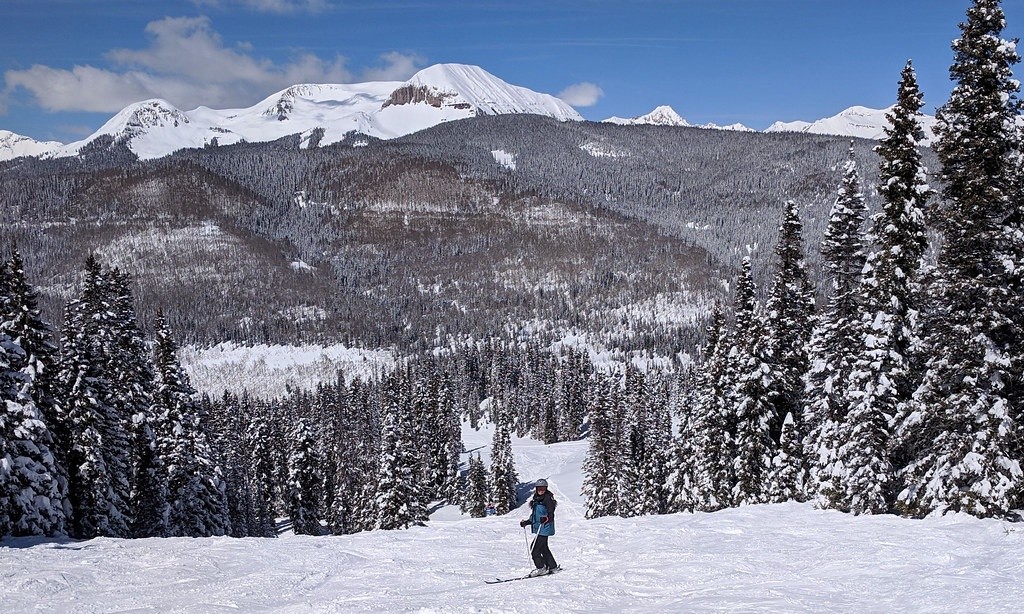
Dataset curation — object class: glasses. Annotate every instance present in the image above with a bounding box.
[537,487,547,492]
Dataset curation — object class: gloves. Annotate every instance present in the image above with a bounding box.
[520,520,532,527]
[540,516,548,524]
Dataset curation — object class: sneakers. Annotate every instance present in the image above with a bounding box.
[546,565,558,573]
[528,565,546,577]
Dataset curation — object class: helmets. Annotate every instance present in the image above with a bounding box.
[534,479,548,487]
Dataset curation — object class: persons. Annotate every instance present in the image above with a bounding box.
[520,478,558,577]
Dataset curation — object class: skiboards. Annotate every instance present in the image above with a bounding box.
[484,564,563,584]
